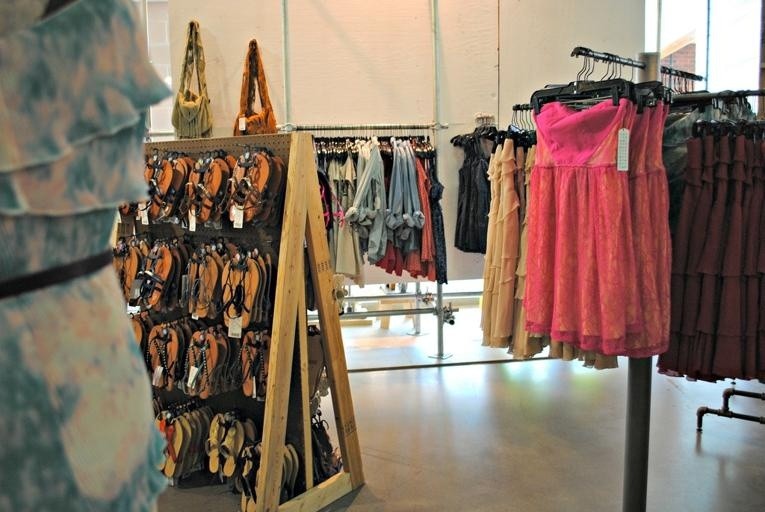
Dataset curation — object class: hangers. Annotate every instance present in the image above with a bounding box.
[530,49,764,137]
[450,105,539,148]
[295,124,437,157]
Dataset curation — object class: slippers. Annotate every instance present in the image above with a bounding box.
[153,402,300,512]
[131,312,271,400]
[119,151,287,229]
[312,422,343,483]
[113,238,272,329]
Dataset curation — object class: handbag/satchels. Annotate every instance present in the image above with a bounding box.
[234,39,276,136]
[172,20,213,137]
